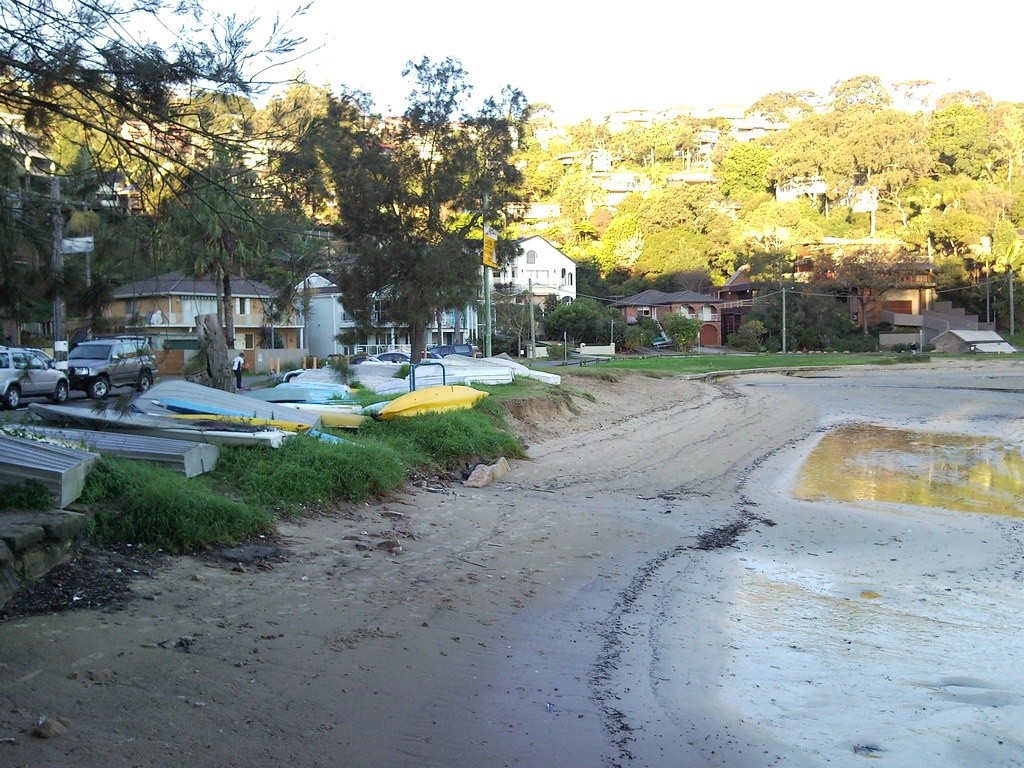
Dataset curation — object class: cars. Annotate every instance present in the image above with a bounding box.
[0,351,69,410]
[349,355,381,365]
[420,350,442,359]
[373,351,411,362]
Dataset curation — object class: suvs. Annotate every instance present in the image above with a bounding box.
[429,343,473,359]
[66,335,159,401]
[0,346,57,370]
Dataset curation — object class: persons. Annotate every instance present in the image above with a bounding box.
[231,353,246,390]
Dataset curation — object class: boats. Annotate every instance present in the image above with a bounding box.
[1,424,221,481]
[29,354,561,449]
[0,434,101,509]
